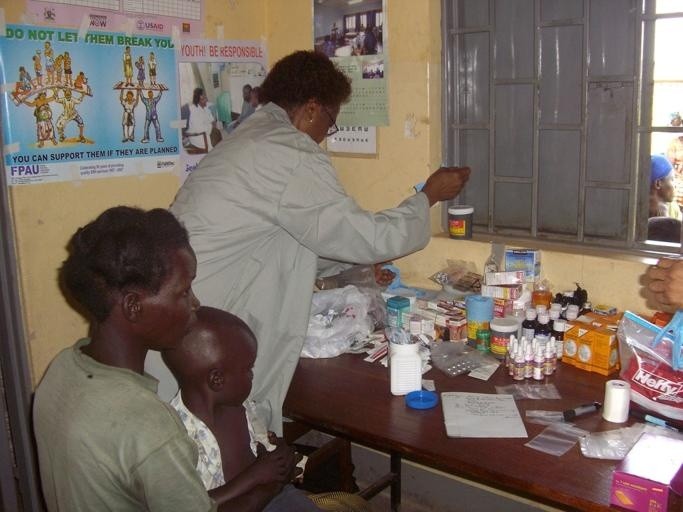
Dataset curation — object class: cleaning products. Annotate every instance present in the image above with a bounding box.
[524,301,580,358]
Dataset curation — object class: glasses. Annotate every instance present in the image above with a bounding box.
[320,105,338,136]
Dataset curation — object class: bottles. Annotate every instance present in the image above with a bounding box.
[447,204,475,240]
[475,282,593,382]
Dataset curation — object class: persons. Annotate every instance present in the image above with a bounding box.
[25,204,372,510]
[226,83,252,132]
[650,155,680,218]
[313,21,382,57]
[179,87,221,155]
[14,42,93,148]
[647,256,683,310]
[248,87,262,111]
[135,49,471,439]
[110,44,168,144]
[162,306,323,512]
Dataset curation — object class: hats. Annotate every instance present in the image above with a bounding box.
[650,155,673,182]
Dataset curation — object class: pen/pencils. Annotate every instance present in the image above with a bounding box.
[563,402,602,420]
[629,409,680,432]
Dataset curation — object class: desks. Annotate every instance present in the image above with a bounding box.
[281,352,683,511]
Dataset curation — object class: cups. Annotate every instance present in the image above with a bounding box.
[386,334,423,397]
